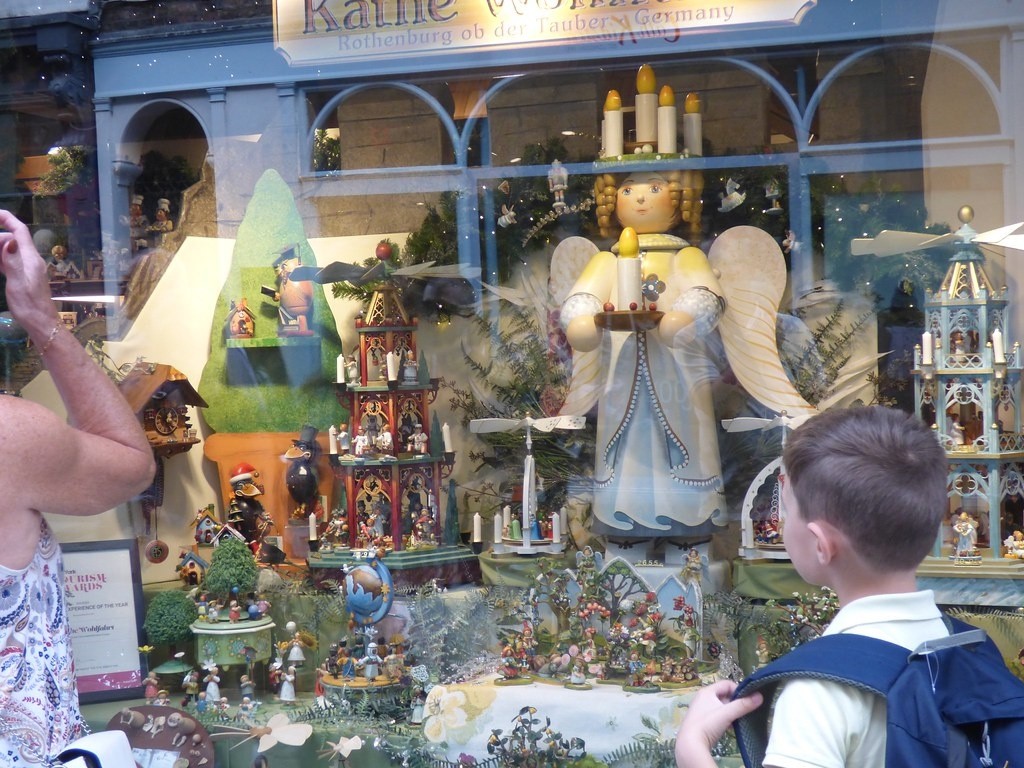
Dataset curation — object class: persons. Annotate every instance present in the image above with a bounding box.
[675,401,1024,768]
[141,349,808,724]
[561,154,728,573]
[953,512,973,559]
[0,211,158,768]
[1011,531,1024,559]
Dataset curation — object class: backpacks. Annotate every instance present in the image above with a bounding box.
[730,613,1024,768]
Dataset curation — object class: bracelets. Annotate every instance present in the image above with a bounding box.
[38,315,63,359]
